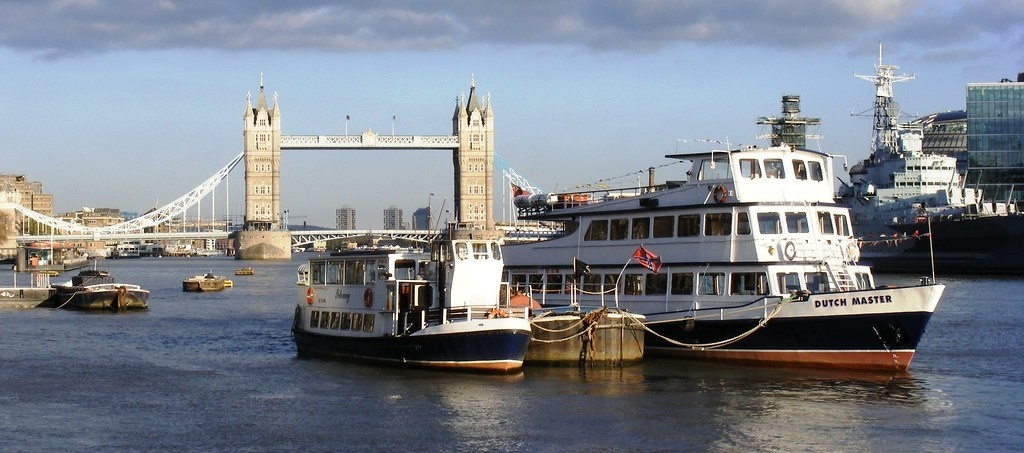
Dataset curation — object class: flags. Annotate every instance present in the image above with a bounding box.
[573,260,590,279]
[511,183,523,197]
[632,244,663,274]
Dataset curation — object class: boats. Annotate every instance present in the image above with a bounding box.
[500,92,947,374]
[181,269,233,293]
[290,192,533,377]
[51,259,151,312]
[836,40,1024,276]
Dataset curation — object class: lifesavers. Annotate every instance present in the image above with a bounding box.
[364,288,373,308]
[306,287,314,305]
[488,310,508,319]
[713,185,728,204]
